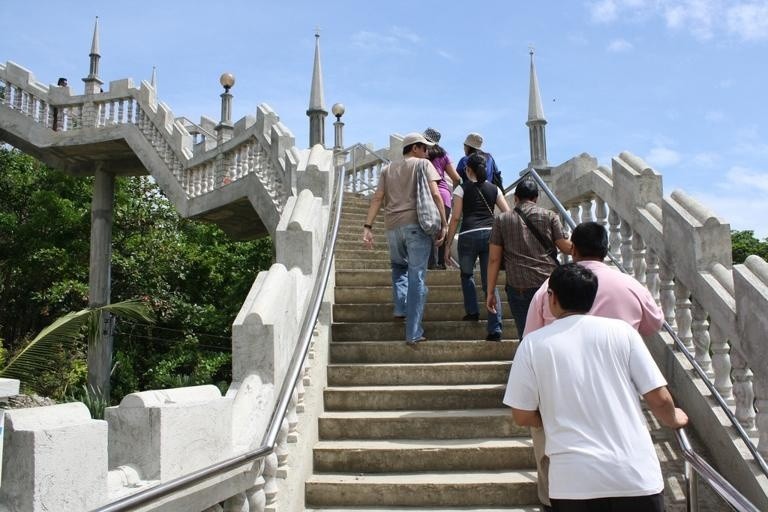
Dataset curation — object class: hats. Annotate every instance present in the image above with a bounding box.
[403,133,436,147]
[464,133,483,149]
[422,128,442,144]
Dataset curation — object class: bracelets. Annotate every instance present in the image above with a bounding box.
[364,224,372,229]
[444,241,452,247]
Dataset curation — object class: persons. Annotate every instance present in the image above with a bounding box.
[454,132,499,184]
[520,220,664,512]
[501,262,690,512]
[52,77,67,131]
[362,132,446,345]
[444,151,512,340]
[486,179,572,342]
[422,127,460,270]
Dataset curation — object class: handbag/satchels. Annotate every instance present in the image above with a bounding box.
[416,160,442,235]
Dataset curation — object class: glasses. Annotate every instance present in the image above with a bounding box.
[464,167,468,172]
[420,145,427,152]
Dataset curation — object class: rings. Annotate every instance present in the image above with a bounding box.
[439,238,442,243]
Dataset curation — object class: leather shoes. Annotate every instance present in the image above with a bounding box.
[487,333,502,341]
[428,263,439,270]
[438,264,446,270]
[406,336,426,346]
[395,316,408,321]
[463,313,479,321]
